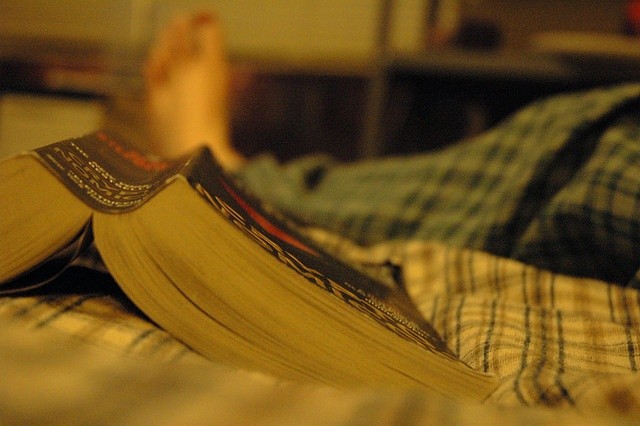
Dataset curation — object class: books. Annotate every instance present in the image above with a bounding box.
[0,125,500,406]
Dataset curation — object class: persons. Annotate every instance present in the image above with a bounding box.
[140,11,639,290]
